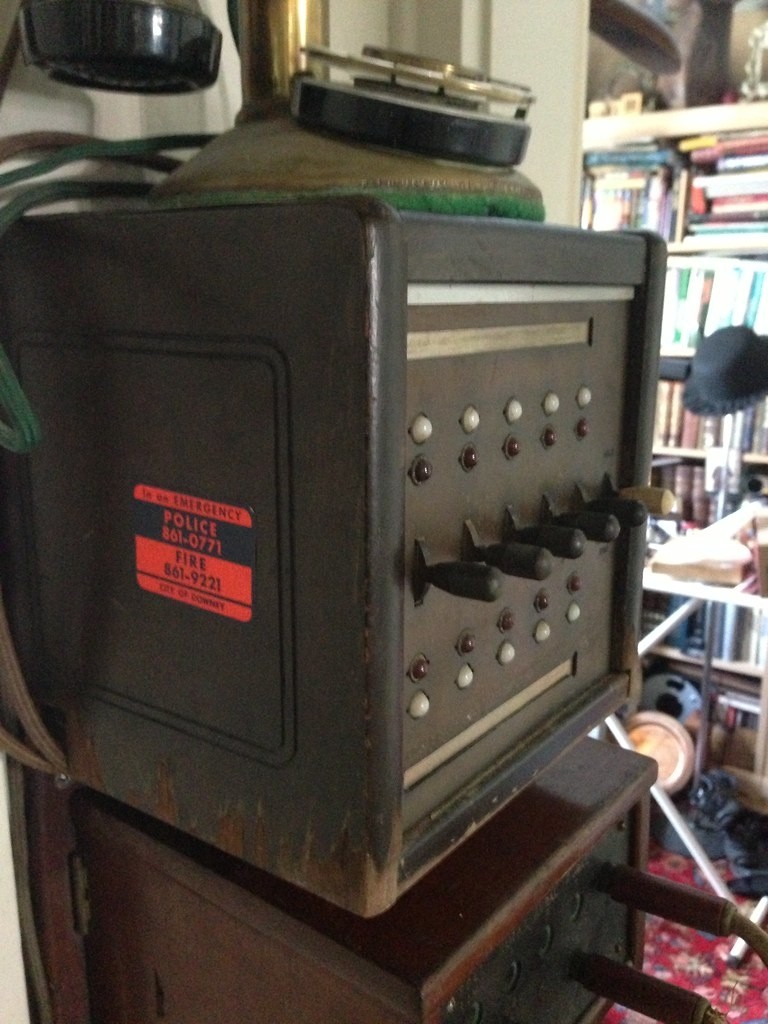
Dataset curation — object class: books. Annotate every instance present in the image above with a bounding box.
[650,384,768,525]
[641,527,768,768]
[581,130,768,251]
[660,255,768,353]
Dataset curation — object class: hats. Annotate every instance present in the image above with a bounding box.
[682,324,768,418]
